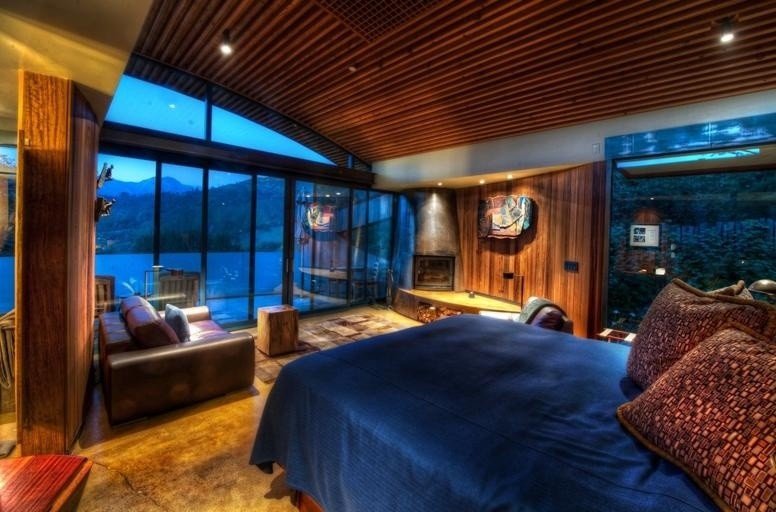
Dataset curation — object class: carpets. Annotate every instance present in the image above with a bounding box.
[254,312,414,384]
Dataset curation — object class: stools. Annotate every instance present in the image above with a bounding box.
[0,455,93,512]
[258,304,299,358]
[594,328,637,346]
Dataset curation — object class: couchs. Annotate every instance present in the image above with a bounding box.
[519,295,574,335]
[98,305,254,427]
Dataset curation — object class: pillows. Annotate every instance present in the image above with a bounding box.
[120,295,154,319]
[627,278,775,391]
[707,280,754,300]
[165,303,190,343]
[615,321,776,512]
[126,305,181,349]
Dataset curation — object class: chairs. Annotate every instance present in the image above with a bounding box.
[159,276,193,310]
[95,278,111,316]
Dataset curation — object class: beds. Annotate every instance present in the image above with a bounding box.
[249,312,776,512]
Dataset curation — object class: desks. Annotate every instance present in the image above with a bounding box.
[393,288,522,323]
[146,291,186,302]
[299,266,357,307]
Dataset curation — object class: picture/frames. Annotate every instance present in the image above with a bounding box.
[628,223,662,251]
[413,254,456,291]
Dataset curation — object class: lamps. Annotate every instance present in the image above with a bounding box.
[719,22,734,43]
[221,28,233,54]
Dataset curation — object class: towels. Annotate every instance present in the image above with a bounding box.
[0,308,15,390]
